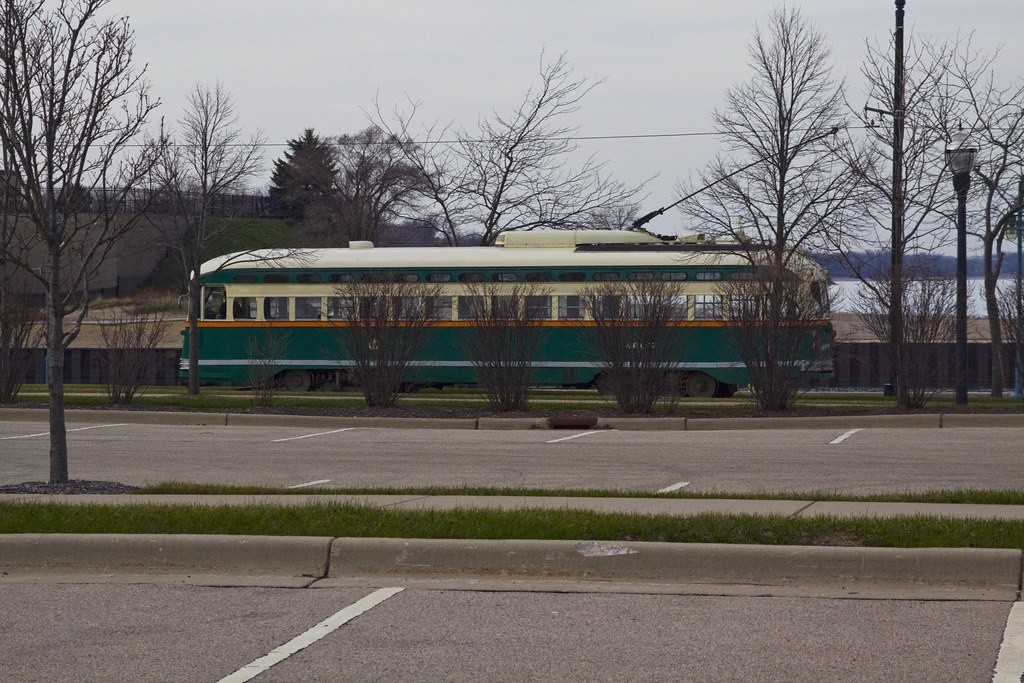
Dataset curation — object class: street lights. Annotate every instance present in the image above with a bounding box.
[945,118,977,405]
[1006,174,1024,399]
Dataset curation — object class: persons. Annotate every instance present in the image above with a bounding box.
[216,290,240,319]
[296,297,318,319]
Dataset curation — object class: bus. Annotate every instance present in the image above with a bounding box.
[179,128,837,398]
